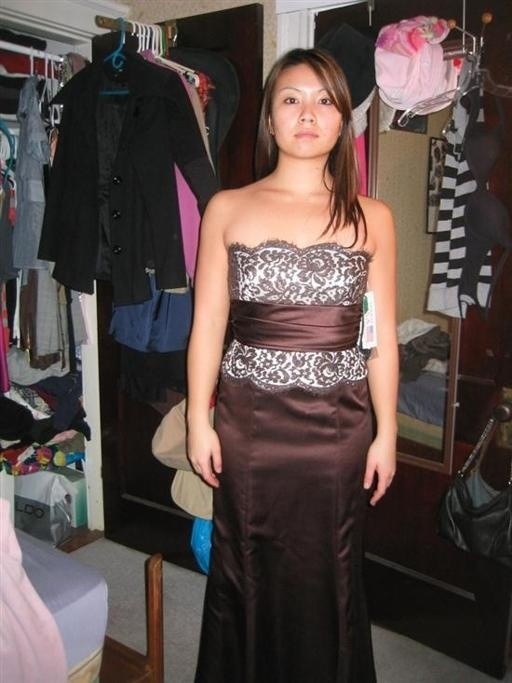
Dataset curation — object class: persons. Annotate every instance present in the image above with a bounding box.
[185,46,400,681]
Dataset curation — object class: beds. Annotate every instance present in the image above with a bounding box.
[0,523,164,682]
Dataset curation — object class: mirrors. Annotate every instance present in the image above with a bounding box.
[368,32,479,476]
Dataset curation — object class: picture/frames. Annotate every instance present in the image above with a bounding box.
[426,136,444,234]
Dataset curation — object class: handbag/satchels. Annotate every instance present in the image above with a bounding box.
[440,468,511,560]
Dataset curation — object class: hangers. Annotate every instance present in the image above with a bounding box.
[16,15,208,127]
[397,45,511,126]
